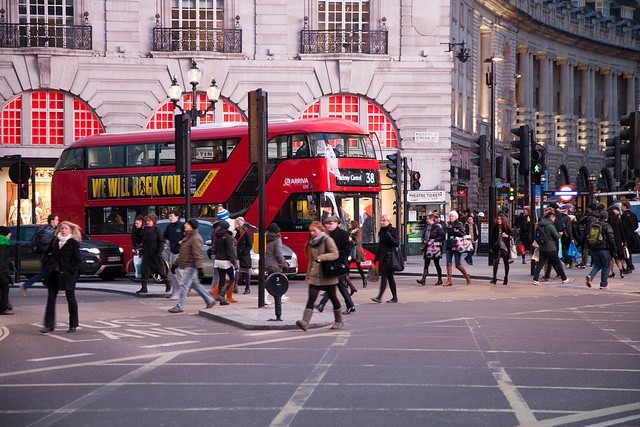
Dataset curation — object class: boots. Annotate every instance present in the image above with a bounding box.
[210,279,219,300]
[443,263,453,287]
[331,307,344,330]
[227,280,238,303]
[296,309,314,332]
[434,268,442,285]
[345,275,357,296]
[264,287,272,305]
[281,293,289,303]
[417,269,428,286]
[455,264,471,285]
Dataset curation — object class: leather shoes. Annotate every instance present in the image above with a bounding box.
[371,298,382,303]
[387,298,398,302]
[1,309,15,315]
[39,325,54,333]
[166,283,171,292]
[18,284,27,298]
[233,287,239,293]
[314,303,324,313]
[243,288,250,294]
[342,306,356,314]
[135,287,148,293]
[468,262,473,266]
[463,257,468,263]
[67,327,77,333]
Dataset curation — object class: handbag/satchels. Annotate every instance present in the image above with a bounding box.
[367,263,379,282]
[488,237,501,261]
[510,236,517,259]
[321,237,349,280]
[349,241,357,259]
[133,250,152,279]
[454,227,474,253]
[41,236,57,266]
[516,239,526,256]
[425,240,442,259]
[384,239,404,272]
[625,211,640,254]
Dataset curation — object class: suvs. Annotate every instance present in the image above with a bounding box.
[6,224,123,279]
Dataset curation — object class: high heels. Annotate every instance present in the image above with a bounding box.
[363,278,368,288]
[608,272,615,278]
[503,277,508,285]
[619,269,625,279]
[490,277,497,284]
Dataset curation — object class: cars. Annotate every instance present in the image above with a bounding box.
[133,218,298,283]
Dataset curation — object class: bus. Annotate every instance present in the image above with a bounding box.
[51,119,386,268]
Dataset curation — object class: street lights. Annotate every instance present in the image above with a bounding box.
[486,54,504,265]
[540,175,547,216]
[169,61,219,218]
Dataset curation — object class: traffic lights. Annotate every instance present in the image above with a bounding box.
[508,183,518,202]
[386,153,400,181]
[606,137,620,178]
[531,149,544,174]
[470,134,487,178]
[457,185,468,196]
[410,171,420,189]
[620,111,640,169]
[19,180,28,198]
[511,124,529,175]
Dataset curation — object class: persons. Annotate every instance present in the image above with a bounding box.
[130,217,144,265]
[135,211,172,293]
[620,201,639,274]
[105,206,125,233]
[567,202,580,266]
[585,210,618,289]
[605,207,628,279]
[19,213,67,297]
[432,209,440,223]
[361,205,372,241]
[373,213,401,303]
[296,220,344,331]
[547,202,576,268]
[162,209,187,269]
[344,273,358,296]
[536,205,562,283]
[168,217,216,313]
[209,228,239,303]
[41,220,83,332]
[457,207,486,221]
[532,211,575,286]
[571,202,600,268]
[264,222,289,305]
[232,215,253,294]
[558,203,575,267]
[347,219,368,288]
[416,213,443,285]
[596,203,606,212]
[443,209,471,286]
[207,206,231,294]
[332,143,343,157]
[312,215,355,314]
[490,214,514,284]
[0,225,15,315]
[514,206,532,264]
[213,218,239,304]
[464,215,478,265]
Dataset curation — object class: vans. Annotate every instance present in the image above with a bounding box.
[606,201,640,248]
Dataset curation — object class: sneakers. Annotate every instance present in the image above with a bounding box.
[562,277,574,284]
[168,306,185,313]
[216,295,226,302]
[600,282,609,289]
[204,299,216,308]
[220,301,229,305]
[586,275,592,287]
[532,280,541,285]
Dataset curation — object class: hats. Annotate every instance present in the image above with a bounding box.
[549,202,559,209]
[323,216,339,222]
[0,226,11,236]
[187,218,199,229]
[235,217,245,227]
[217,206,230,220]
[448,209,458,219]
[267,223,281,233]
[559,204,569,212]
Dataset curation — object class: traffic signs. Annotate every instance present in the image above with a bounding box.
[449,166,470,181]
[558,184,575,202]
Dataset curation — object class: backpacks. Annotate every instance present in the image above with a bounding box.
[27,224,48,258]
[535,222,549,246]
[586,222,609,247]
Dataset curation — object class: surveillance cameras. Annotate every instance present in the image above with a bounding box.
[456,48,470,63]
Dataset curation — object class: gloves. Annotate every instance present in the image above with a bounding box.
[170,263,178,275]
[197,269,204,279]
[234,262,238,271]
